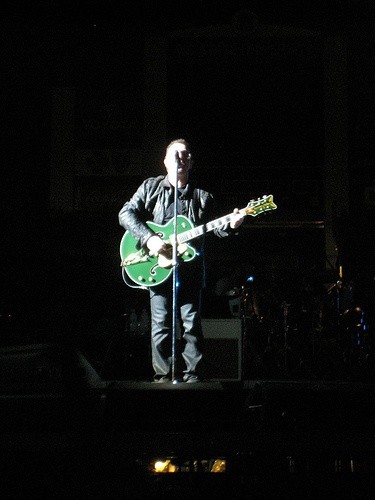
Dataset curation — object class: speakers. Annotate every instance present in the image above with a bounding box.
[176,319,242,383]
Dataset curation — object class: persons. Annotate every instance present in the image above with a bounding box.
[119,139,243,384]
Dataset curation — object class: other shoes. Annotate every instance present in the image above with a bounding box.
[152,379,169,383]
[186,376,198,383]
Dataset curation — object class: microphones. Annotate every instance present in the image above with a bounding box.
[173,150,182,167]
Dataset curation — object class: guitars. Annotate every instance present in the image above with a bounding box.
[120,195,277,286]
